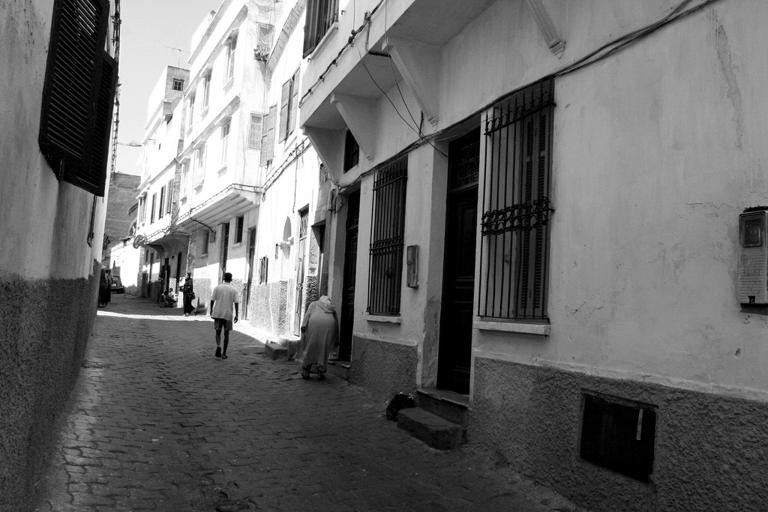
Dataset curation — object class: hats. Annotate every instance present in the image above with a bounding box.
[223,273,232,280]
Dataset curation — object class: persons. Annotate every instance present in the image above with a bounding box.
[97,265,114,308]
[182,272,196,317]
[158,288,178,308]
[209,272,240,359]
[299,295,340,381]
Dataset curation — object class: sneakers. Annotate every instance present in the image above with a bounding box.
[300,372,324,381]
[216,347,228,359]
